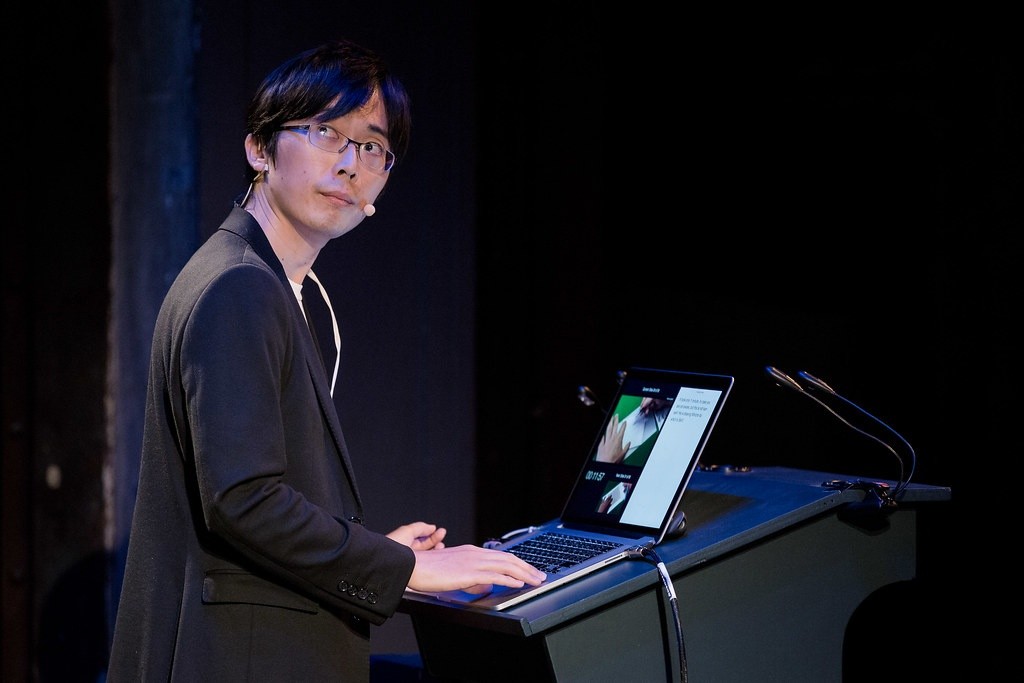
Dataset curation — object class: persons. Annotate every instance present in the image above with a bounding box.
[118,39,546,683]
[596,413,631,463]
[598,495,613,514]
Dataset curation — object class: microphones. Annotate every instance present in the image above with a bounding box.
[364,204,375,216]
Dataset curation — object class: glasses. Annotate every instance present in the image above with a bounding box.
[278,123,396,172]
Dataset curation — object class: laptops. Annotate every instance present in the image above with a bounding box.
[404,368,736,610]
